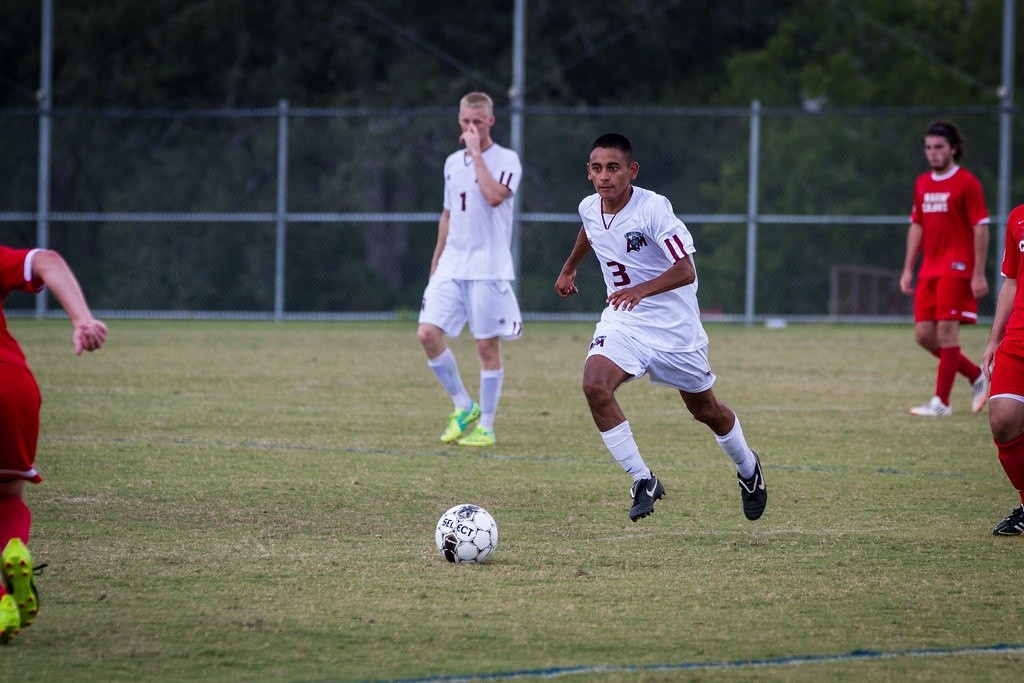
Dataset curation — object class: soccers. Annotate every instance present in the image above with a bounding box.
[435,504,499,563]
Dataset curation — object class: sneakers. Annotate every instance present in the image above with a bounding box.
[971,364,992,414]
[0,594,21,644]
[0,538,48,627]
[458,425,496,447]
[629,471,666,522]
[993,505,1024,537]
[910,396,952,416]
[737,449,767,521]
[441,401,480,443]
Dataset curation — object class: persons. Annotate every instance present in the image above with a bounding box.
[0,248,107,643]
[900,124,992,415]
[556,134,767,522]
[417,92,522,446]
[983,204,1024,535]
